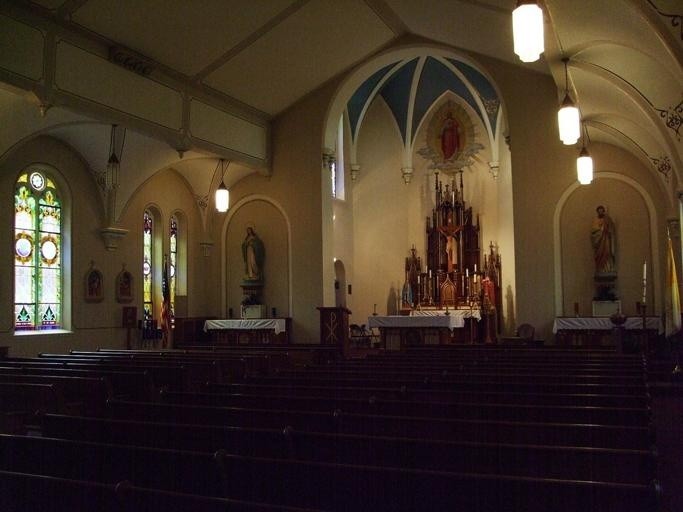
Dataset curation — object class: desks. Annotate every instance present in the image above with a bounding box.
[553,318,662,349]
[203,318,287,341]
[366,316,465,347]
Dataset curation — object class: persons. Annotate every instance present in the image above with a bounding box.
[160,301,173,347]
[439,111,460,160]
[591,205,617,273]
[241,227,266,278]
[404,248,421,288]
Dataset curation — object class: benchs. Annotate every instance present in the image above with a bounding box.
[0,344,681,511]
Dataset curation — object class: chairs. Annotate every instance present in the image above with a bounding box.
[517,324,534,343]
[350,324,380,344]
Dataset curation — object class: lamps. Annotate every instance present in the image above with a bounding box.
[576,124,594,186]
[215,157,230,212]
[512,1,546,63]
[557,54,581,146]
[106,123,121,190]
